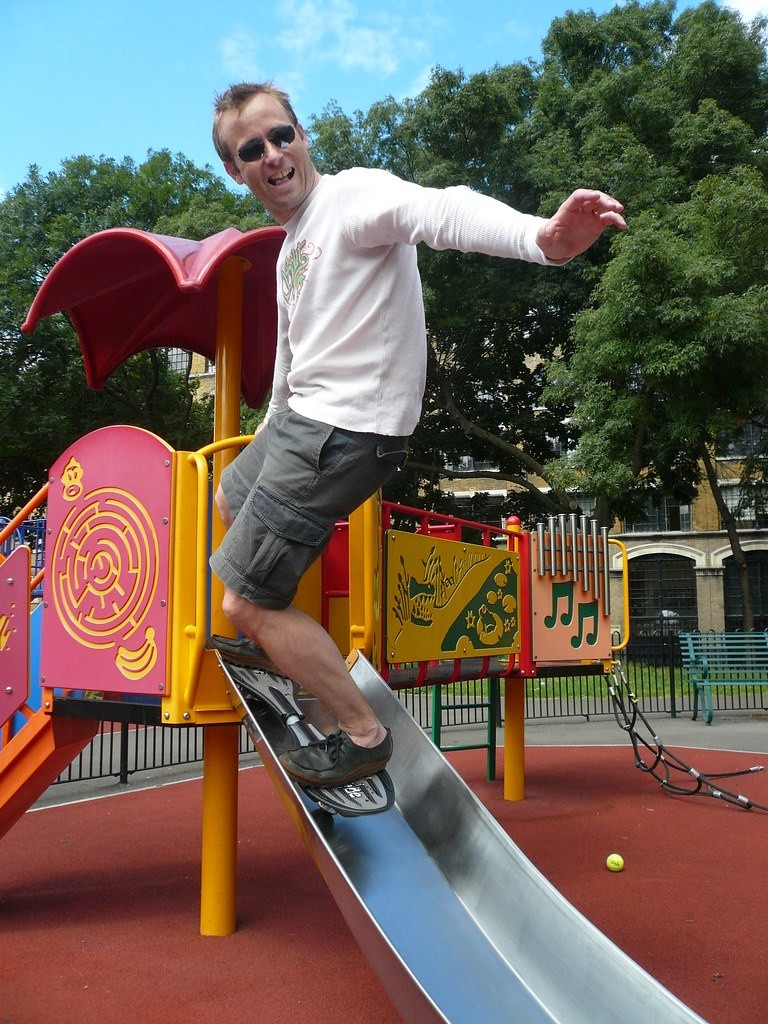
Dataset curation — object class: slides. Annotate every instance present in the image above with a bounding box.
[202,645,715,1024]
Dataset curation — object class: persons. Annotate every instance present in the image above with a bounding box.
[208,80,627,789]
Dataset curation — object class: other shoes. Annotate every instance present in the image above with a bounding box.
[207,633,291,678]
[277,725,393,788]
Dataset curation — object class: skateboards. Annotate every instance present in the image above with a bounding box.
[223,661,397,817]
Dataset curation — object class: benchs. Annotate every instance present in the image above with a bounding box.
[677,631,768,723]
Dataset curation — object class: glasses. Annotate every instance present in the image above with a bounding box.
[228,126,296,163]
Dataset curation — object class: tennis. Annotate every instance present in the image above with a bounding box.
[606,852,625,873]
